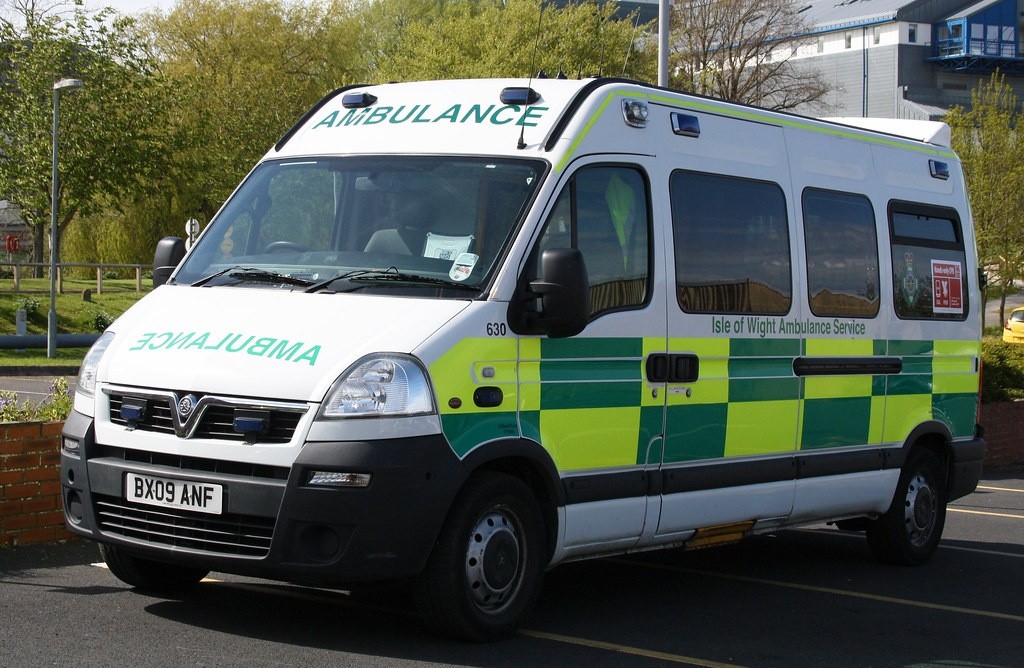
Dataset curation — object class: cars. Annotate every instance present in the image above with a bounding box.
[1003,306,1024,344]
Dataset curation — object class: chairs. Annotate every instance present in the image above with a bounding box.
[541,191,624,277]
[364,190,435,256]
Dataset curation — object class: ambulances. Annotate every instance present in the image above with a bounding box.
[58,69,987,643]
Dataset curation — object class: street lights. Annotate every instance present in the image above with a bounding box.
[46,76,82,359]
